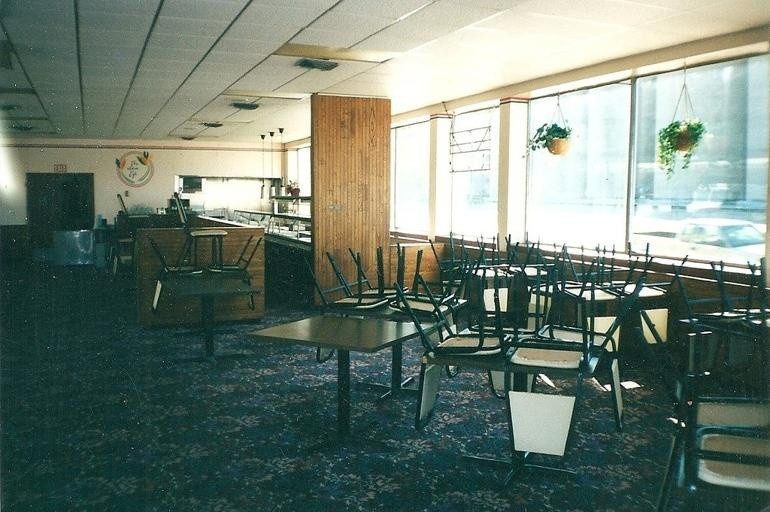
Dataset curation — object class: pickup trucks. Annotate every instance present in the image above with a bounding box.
[629,217,766,268]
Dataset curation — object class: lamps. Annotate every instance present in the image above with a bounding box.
[260,128,286,201]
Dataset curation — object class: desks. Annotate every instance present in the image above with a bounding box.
[248,314,442,455]
[159,270,265,370]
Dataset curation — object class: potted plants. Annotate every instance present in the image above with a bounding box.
[519,114,573,159]
[285,179,300,198]
[658,116,707,177]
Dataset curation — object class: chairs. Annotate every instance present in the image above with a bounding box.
[51,228,98,298]
[145,192,263,313]
